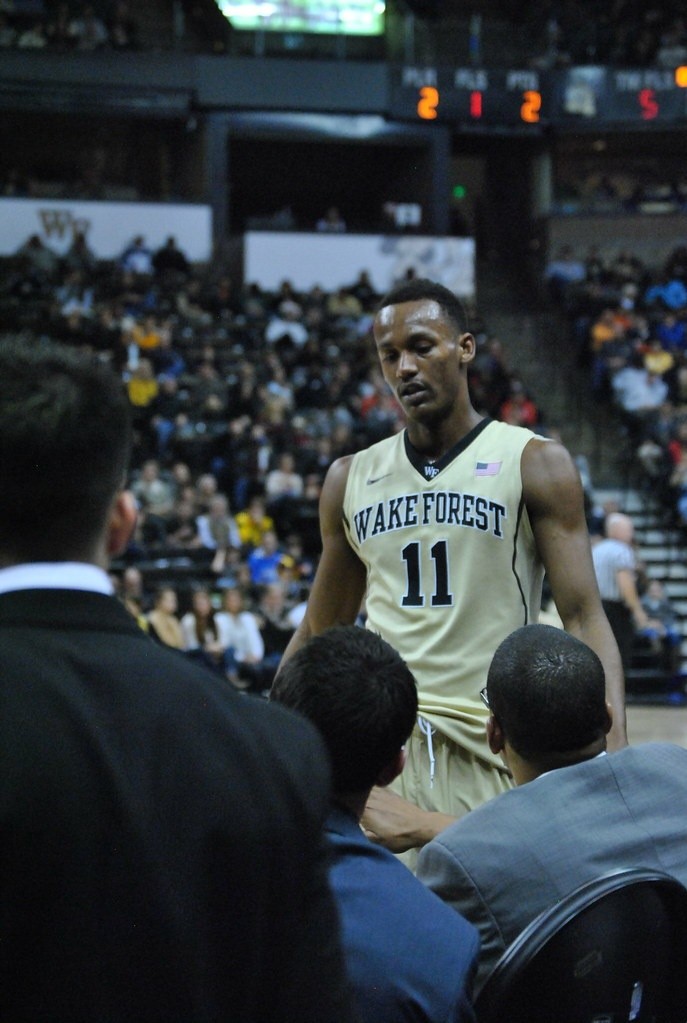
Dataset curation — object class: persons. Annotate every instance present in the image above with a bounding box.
[0,203,687,693]
[361,624,687,998]
[0,339,344,1023]
[273,624,484,1023]
[268,282,623,860]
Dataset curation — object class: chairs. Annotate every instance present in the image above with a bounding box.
[473,867,687,1022]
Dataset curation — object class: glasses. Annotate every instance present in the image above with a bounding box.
[480,688,490,709]
